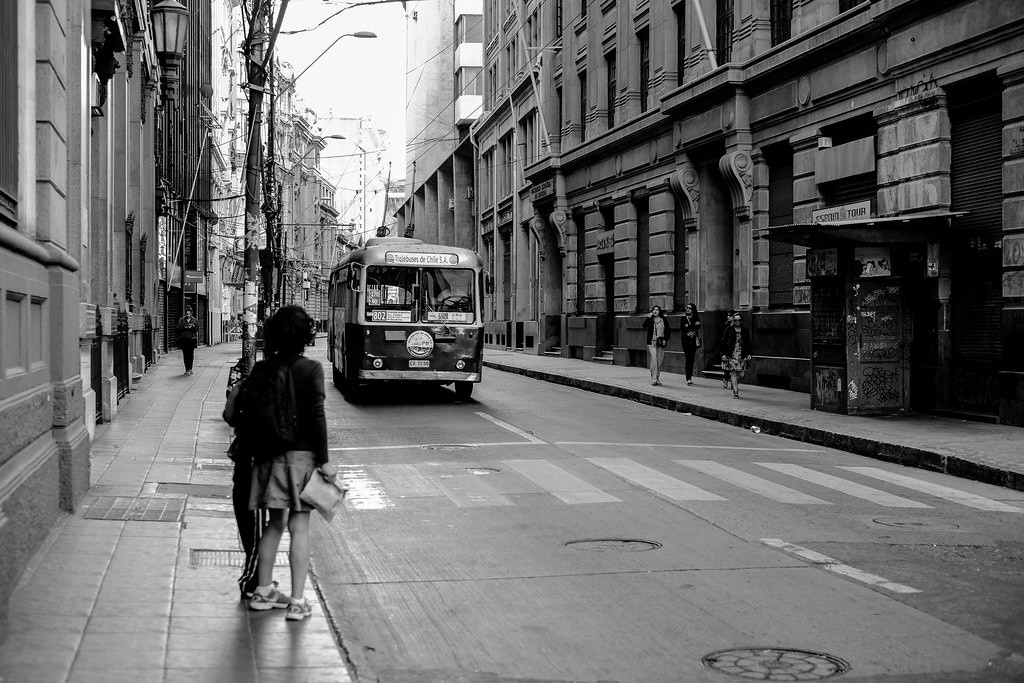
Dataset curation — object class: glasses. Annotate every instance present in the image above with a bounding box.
[733,319,742,322]
[685,306,692,310]
[727,314,733,317]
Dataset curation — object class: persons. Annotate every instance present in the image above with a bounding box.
[223,306,337,621]
[680,303,701,384]
[721,310,752,399]
[437,278,468,312]
[177,306,199,376]
[642,305,671,385]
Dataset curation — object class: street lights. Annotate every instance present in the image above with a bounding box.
[282,196,332,305]
[265,30,378,316]
[275,133,344,307]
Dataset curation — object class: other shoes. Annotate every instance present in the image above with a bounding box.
[241,581,278,601]
[723,378,728,387]
[733,391,739,399]
[657,380,662,385]
[651,379,657,385]
[686,380,693,386]
[184,369,194,375]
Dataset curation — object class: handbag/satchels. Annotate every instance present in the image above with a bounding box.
[696,336,700,347]
[656,337,668,348]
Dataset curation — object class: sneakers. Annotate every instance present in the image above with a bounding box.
[250,588,291,610]
[285,597,313,620]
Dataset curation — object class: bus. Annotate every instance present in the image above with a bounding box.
[325,235,494,400]
